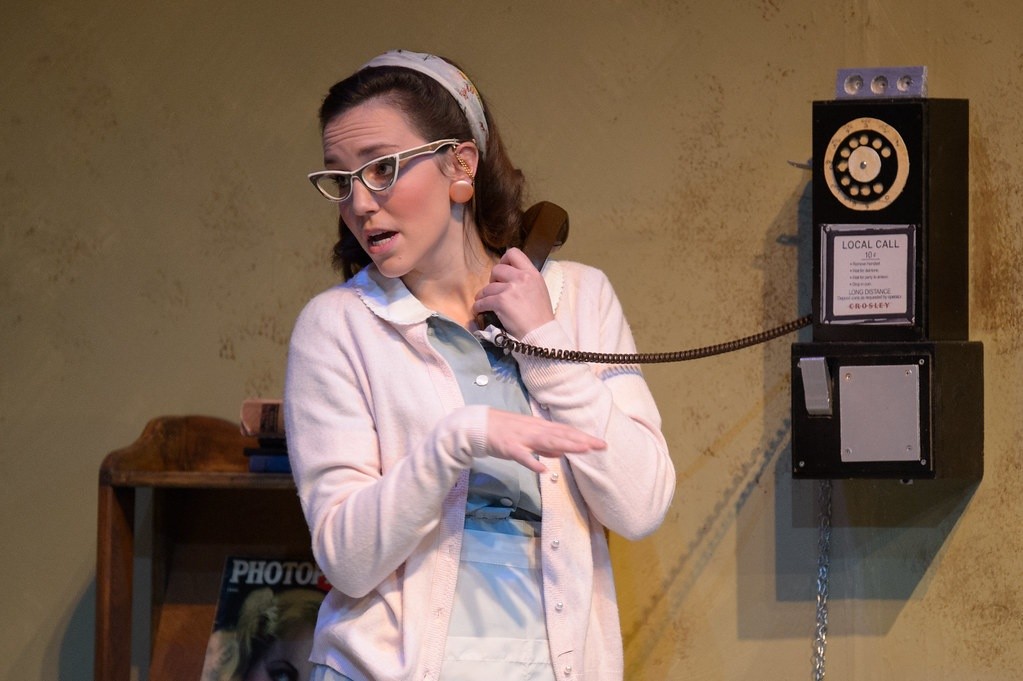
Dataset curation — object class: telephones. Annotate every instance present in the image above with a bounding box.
[477,202,570,334]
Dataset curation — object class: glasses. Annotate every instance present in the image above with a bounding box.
[306,139,461,202]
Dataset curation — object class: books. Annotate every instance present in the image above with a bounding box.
[235,399,293,473]
[200,555,332,681]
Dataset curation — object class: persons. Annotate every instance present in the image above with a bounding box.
[218,588,326,681]
[280,49,676,681]
